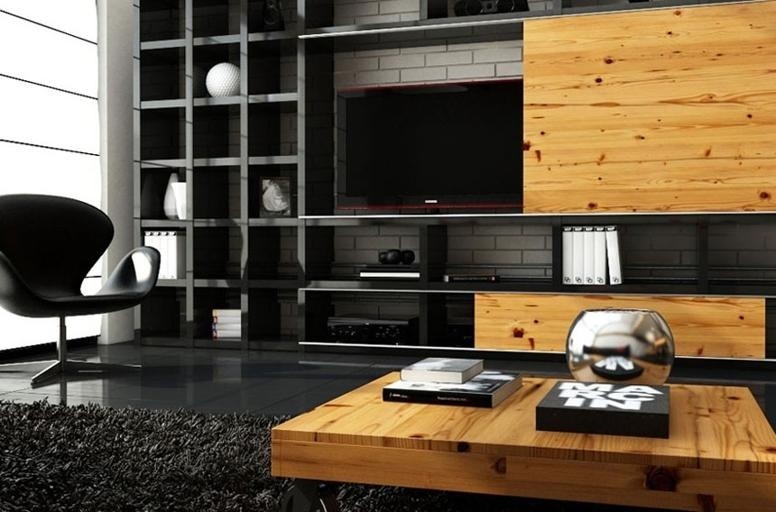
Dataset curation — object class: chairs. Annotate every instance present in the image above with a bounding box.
[0,195,164,386]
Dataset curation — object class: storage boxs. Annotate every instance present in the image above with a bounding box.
[142,229,187,281]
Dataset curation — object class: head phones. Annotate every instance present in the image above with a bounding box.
[379,249,415,264]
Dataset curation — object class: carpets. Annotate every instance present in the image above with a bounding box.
[1,403,459,511]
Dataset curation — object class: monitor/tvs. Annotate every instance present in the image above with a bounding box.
[336,80,523,214]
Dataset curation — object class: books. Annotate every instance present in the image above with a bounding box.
[211,308,242,338]
[400,357,484,384]
[537,382,672,435]
[142,230,185,279]
[383,368,522,408]
[560,226,623,284]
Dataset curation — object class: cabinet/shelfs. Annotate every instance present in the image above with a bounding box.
[302,0,776,365]
[129,0,335,355]
